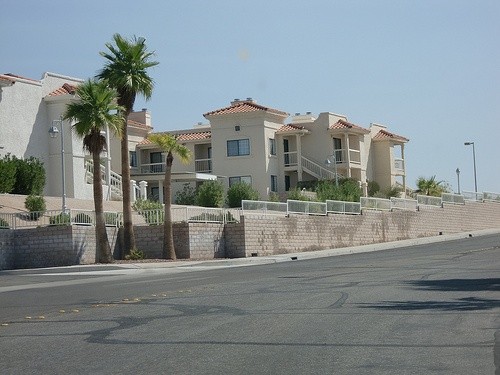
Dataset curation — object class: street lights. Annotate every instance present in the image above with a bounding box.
[463,141,479,200]
[50,117,67,212]
[456,168,462,195]
